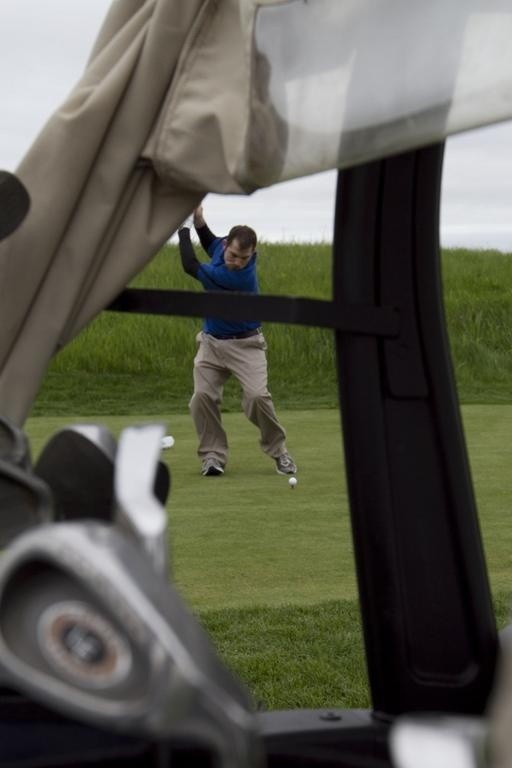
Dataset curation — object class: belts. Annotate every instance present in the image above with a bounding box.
[208,327,262,340]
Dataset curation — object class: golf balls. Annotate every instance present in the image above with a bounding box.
[289,477,297,486]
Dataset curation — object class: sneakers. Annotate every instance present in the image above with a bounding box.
[273,453,298,476]
[199,459,225,477]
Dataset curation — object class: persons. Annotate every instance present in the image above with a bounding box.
[175,199,298,477]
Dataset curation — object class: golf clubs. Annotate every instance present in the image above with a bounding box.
[0,418,171,550]
[0,519,260,768]
[108,422,170,580]
